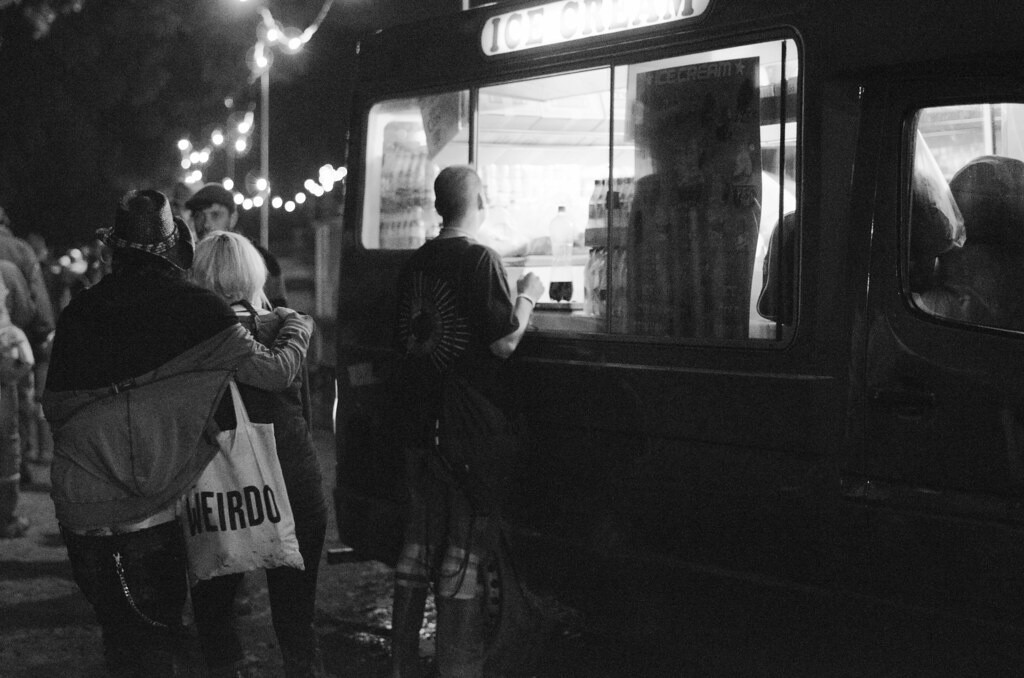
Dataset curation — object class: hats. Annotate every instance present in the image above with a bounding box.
[184,184,234,212]
[97,189,197,271]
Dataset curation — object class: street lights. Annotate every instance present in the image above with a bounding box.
[246,19,307,252]
[201,110,255,196]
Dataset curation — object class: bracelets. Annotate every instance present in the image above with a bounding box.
[516,293,535,308]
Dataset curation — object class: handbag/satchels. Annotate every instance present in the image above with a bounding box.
[177,373,306,581]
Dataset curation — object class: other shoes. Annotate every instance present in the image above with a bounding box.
[1,514,28,539]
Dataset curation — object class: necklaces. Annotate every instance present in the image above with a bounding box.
[444,226,462,231]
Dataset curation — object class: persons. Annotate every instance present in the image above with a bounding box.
[389,168,537,678]
[189,231,328,678]
[41,189,314,678]
[935,156,1024,332]
[186,183,289,308]
[0,206,112,537]
